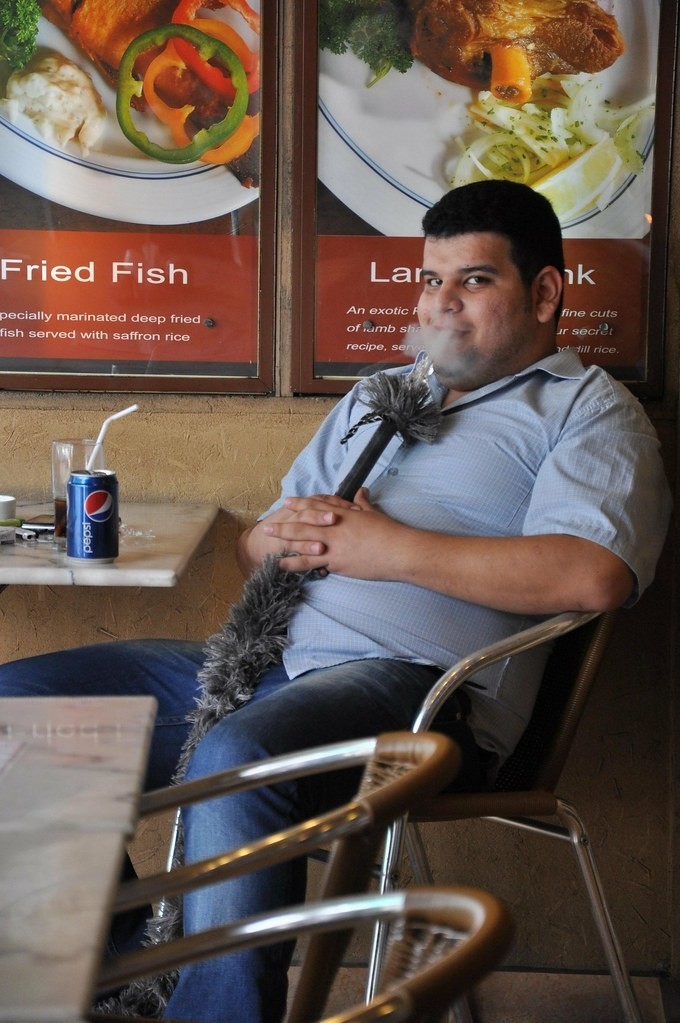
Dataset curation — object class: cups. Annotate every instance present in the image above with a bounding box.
[51,438,105,554]
[0,495,17,522]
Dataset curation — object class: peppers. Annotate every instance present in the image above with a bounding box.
[115,0,262,165]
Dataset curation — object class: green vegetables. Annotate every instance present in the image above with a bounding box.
[318,0,414,88]
[0,0,42,70]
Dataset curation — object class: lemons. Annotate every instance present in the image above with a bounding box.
[526,142,624,222]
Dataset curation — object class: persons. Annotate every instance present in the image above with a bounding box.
[0,178,669,1023]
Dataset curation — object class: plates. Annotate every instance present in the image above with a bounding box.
[318,1,664,238]
[1,0,263,226]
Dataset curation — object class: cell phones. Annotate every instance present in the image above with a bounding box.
[20,514,55,534]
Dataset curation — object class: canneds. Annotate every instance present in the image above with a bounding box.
[66,469,120,564]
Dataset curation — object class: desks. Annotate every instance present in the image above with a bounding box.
[0,694,161,1023]
[2,500,226,588]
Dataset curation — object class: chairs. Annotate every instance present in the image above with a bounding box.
[88,594,625,1023]
